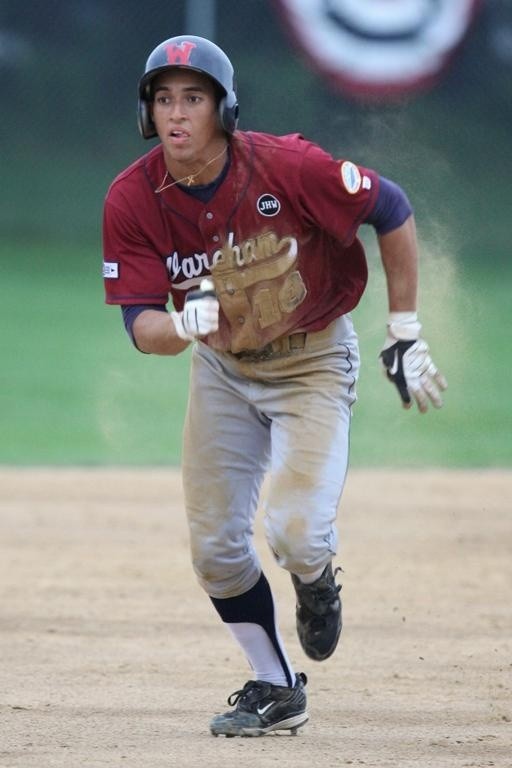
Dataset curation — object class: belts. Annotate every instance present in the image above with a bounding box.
[243,327,338,359]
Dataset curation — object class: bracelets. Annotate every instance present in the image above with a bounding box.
[386,311,422,340]
[170,311,194,341]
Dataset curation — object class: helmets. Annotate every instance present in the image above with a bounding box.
[137,35,240,139]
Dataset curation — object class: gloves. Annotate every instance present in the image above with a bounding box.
[171,279,219,342]
[380,310,447,413]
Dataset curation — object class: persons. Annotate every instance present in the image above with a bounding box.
[105,34,447,738]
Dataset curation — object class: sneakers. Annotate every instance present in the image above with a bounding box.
[291,561,343,662]
[209,671,310,737]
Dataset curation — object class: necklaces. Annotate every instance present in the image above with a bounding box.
[152,146,230,194]
